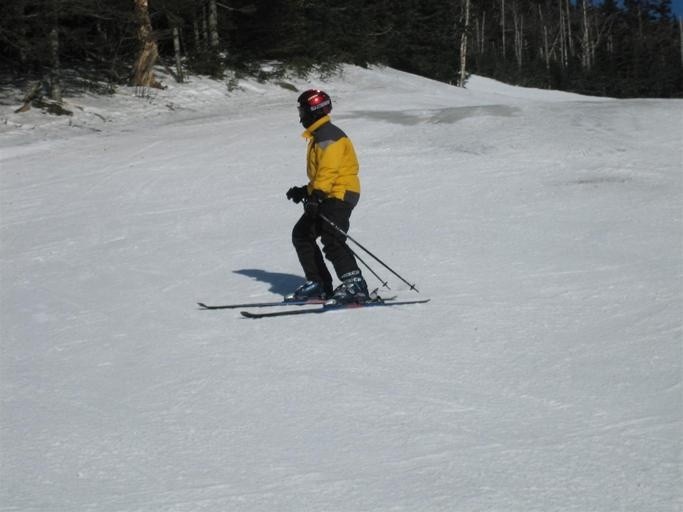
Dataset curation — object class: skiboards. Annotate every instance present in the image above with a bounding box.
[196,286,432,319]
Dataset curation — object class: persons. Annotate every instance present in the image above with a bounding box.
[286,88,369,307]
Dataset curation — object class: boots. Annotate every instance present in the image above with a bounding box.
[332,270,370,303]
[295,279,334,299]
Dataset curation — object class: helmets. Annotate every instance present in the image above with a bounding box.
[297,88,332,116]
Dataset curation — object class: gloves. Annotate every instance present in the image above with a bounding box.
[304,195,323,216]
[291,185,308,204]
